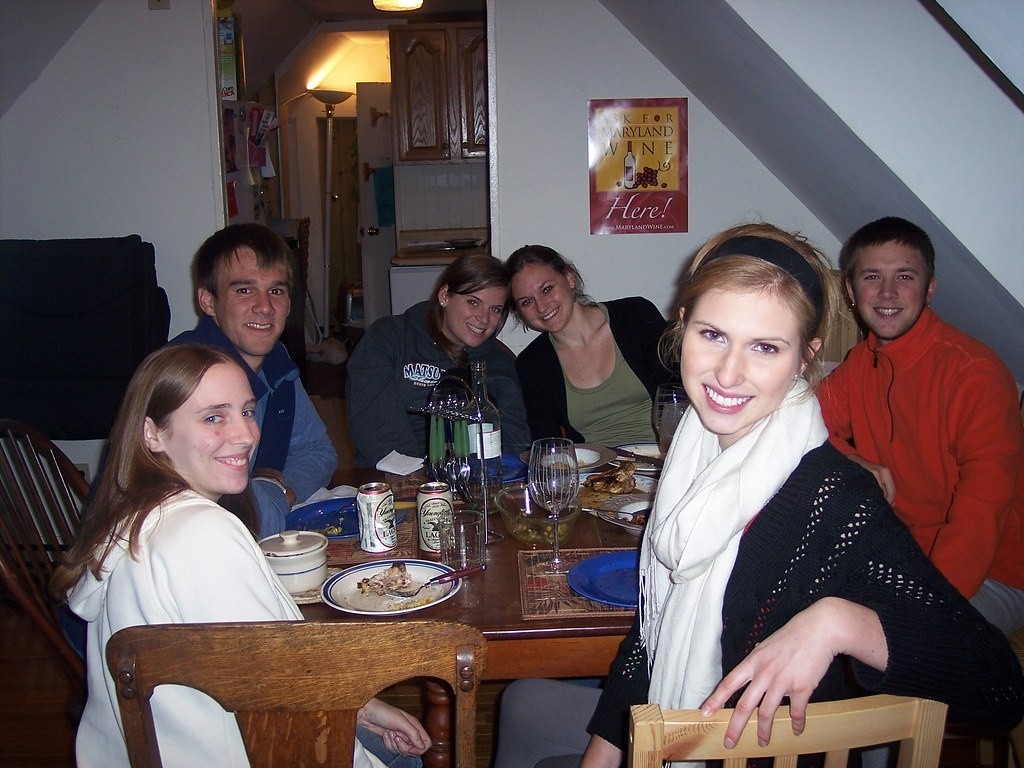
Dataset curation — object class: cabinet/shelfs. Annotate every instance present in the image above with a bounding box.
[389,23,489,165]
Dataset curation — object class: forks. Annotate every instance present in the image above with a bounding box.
[382,564,487,598]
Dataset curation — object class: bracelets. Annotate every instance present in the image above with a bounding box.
[252,475,287,496]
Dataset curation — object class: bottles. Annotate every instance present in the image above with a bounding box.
[465,361,503,500]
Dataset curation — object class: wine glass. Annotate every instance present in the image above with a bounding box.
[528,438,579,573]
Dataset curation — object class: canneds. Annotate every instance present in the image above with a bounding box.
[357,482,397,554]
[416,482,455,552]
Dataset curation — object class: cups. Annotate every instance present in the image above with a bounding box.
[653,385,688,435]
[659,403,688,455]
[439,510,486,572]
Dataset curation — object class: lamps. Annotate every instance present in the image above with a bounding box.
[308,90,355,340]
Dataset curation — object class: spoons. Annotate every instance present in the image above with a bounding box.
[433,462,472,500]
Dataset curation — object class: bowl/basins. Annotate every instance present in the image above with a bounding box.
[597,492,656,537]
[519,442,616,472]
[256,530,328,594]
[494,485,581,548]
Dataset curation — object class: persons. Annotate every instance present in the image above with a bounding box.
[506,244,692,449]
[813,214,1024,637]
[342,253,535,476]
[49,343,433,768]
[168,221,339,543]
[578,223,1024,768]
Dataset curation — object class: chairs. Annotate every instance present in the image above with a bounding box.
[0,412,950,768]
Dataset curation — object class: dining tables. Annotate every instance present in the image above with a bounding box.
[254,445,641,768]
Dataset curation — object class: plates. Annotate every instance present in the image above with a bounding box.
[607,442,661,476]
[286,497,407,540]
[555,473,658,509]
[321,559,461,616]
[468,453,528,483]
[566,551,638,607]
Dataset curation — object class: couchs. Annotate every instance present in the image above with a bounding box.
[0,234,173,438]
